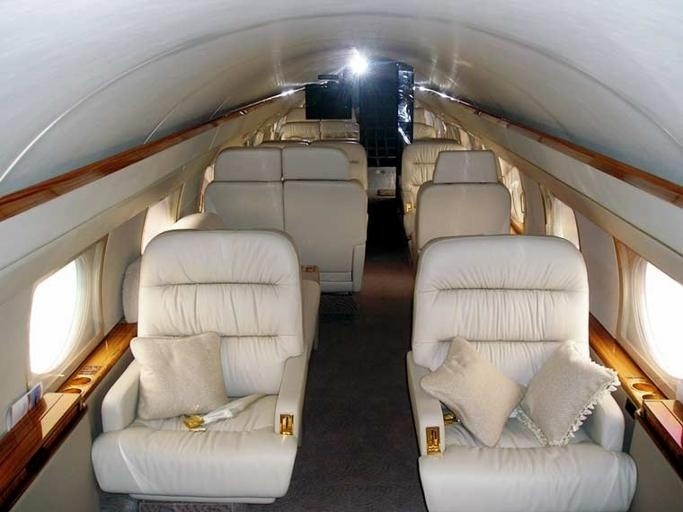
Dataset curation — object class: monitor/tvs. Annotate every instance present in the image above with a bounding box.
[305,84,351,119]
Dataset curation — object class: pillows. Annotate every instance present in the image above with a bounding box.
[419,336,529,447]
[515,341,620,446]
[130,333,229,419]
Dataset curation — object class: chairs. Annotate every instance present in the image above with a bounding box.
[415,150,511,262]
[199,122,365,293]
[400,140,469,253]
[93,227,309,504]
[124,214,321,325]
[407,235,639,510]
